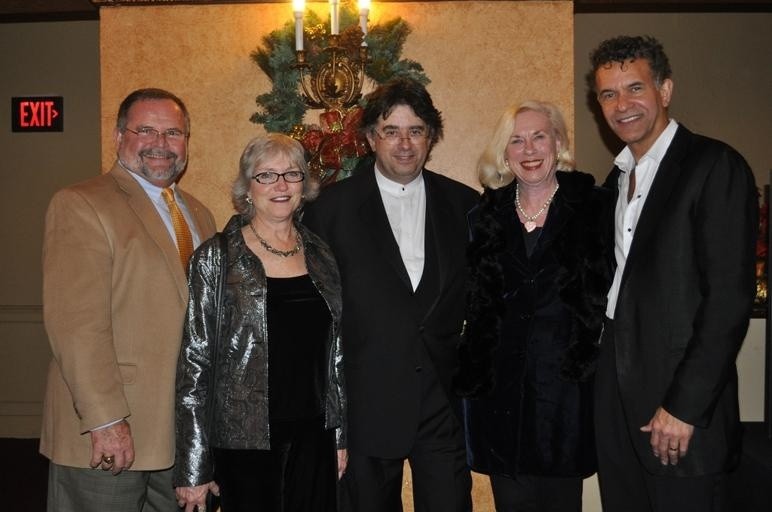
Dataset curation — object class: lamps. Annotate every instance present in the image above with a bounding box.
[290,0,371,109]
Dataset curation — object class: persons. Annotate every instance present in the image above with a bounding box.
[37,87,216,511]
[452,98,619,512]
[581,32,760,510]
[171,132,351,512]
[299,76,485,512]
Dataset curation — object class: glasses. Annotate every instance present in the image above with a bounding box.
[125,128,189,141]
[252,171,305,184]
[374,128,431,145]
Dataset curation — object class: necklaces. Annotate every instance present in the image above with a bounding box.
[246,218,302,259]
[514,178,560,234]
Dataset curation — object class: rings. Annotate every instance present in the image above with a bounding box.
[196,506,206,512]
[669,445,679,452]
[102,454,115,464]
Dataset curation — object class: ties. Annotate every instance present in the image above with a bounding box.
[161,187,194,279]
